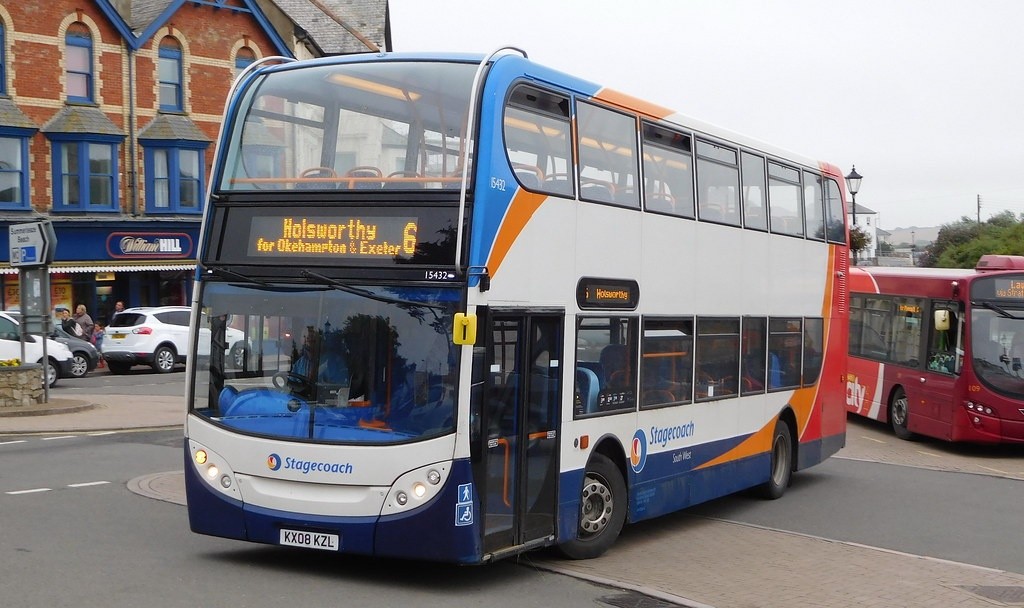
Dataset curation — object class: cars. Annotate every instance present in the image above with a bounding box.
[0,310,101,391]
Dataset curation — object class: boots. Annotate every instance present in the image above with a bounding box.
[98,359,105,368]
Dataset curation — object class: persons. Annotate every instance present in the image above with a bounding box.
[289,325,349,386]
[61,305,106,368]
[112,301,124,319]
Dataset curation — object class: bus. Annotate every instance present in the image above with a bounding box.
[183,45,851,568]
[846,255,1024,446]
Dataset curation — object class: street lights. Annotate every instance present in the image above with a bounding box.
[844,164,864,266]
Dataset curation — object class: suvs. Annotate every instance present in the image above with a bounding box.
[101,306,252,374]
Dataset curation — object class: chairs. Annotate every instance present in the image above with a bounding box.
[295,166,471,190]
[609,370,753,403]
[512,161,785,234]
